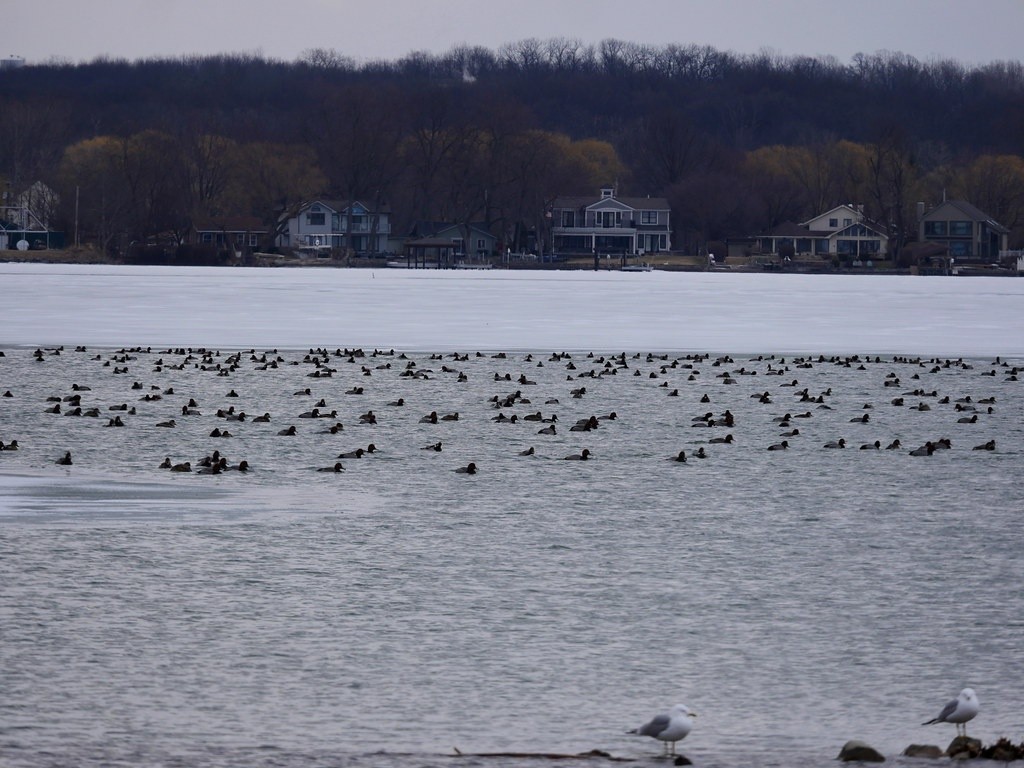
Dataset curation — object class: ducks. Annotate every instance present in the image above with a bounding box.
[0,344,1023,476]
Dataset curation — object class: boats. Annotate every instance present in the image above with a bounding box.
[622,258,655,272]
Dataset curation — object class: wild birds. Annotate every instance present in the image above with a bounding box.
[923,688,982,740]
[625,701,697,759]
